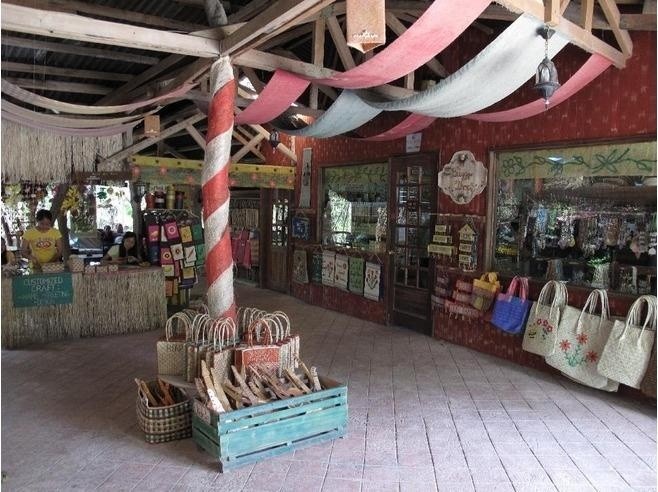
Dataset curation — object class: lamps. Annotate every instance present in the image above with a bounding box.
[532,25,562,108]
[268,128,282,154]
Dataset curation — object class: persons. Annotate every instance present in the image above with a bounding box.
[100,231,143,266]
[101,225,114,243]
[113,224,125,243]
[1,237,16,266]
[21,210,63,267]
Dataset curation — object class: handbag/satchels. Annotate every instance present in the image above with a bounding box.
[522,280,657,393]
[292,249,383,302]
[431,265,532,335]
[231,228,259,269]
[157,304,300,383]
[148,222,205,297]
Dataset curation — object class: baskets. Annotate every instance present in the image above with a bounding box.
[96,265,107,273]
[84,265,96,273]
[109,265,118,272]
[39,262,65,272]
[136,379,193,444]
[67,255,84,273]
